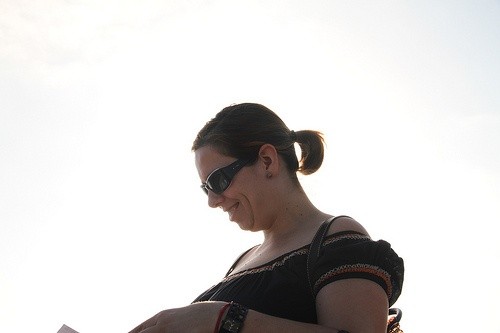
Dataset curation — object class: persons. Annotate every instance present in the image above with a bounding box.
[126,103,404,333]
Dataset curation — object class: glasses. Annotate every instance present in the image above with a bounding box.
[200,160,248,194]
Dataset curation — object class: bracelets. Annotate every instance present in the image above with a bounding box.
[214,303,230,332]
[221,300,249,333]
